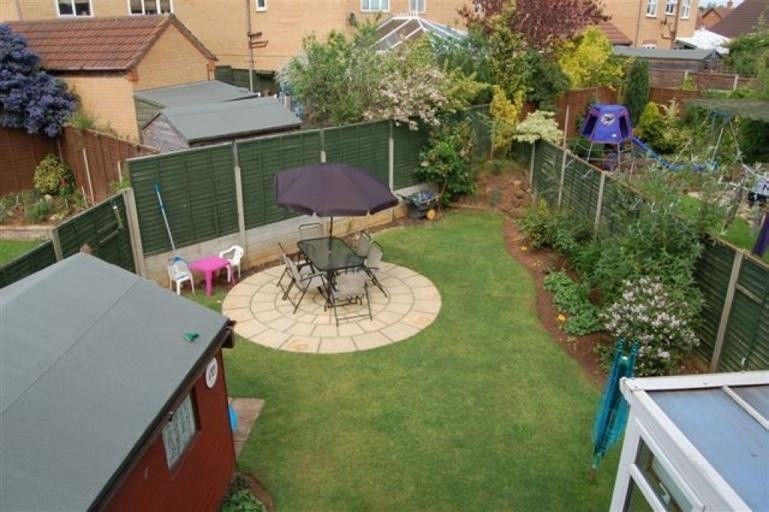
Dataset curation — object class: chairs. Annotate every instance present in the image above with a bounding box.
[216,244,245,281]
[167,255,197,295]
[277,222,387,327]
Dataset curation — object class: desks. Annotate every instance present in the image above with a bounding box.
[187,255,236,296]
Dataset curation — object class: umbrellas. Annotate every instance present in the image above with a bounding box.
[273,161,399,255]
